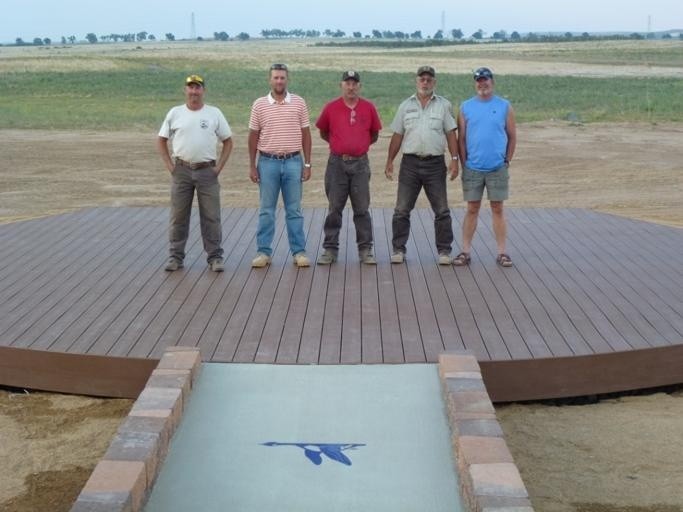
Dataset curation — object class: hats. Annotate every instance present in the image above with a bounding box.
[474,67,492,80]
[186,75,204,86]
[342,72,360,82]
[418,66,435,76]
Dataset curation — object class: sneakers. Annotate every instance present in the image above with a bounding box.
[166,258,184,271]
[252,253,271,267]
[359,249,404,264]
[439,253,471,265]
[208,259,225,272]
[293,247,337,266]
[497,254,512,267]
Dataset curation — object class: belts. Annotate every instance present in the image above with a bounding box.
[178,158,216,169]
[420,155,441,161]
[259,151,300,159]
[339,155,361,161]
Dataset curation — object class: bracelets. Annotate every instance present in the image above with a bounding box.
[450,156,458,160]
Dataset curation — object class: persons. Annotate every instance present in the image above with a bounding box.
[383,66,457,265]
[450,68,514,267]
[158,72,234,271]
[315,70,381,264]
[246,63,312,268]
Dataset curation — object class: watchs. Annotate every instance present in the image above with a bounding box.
[304,164,310,167]
[503,159,511,165]
